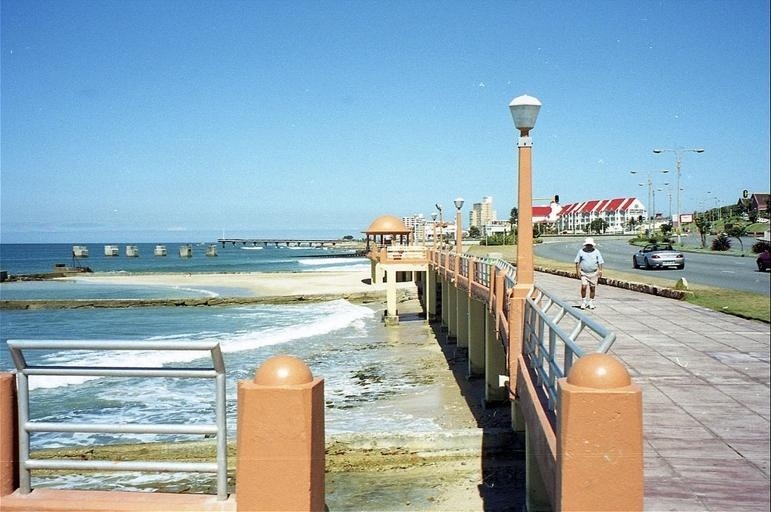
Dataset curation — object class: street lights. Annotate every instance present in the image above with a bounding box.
[629,146,725,244]
[453,195,466,255]
[505,90,546,289]
[515,218,518,225]
[557,219,560,235]
[430,211,440,251]
[421,218,427,246]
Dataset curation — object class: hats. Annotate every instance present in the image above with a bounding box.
[583,238,596,247]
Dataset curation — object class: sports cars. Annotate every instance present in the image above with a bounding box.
[631,243,685,270]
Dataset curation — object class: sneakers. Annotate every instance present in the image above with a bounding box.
[581,302,595,309]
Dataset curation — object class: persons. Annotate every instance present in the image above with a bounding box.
[574,237,606,310]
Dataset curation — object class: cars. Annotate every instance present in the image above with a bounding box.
[754,248,771,272]
[751,238,771,253]
